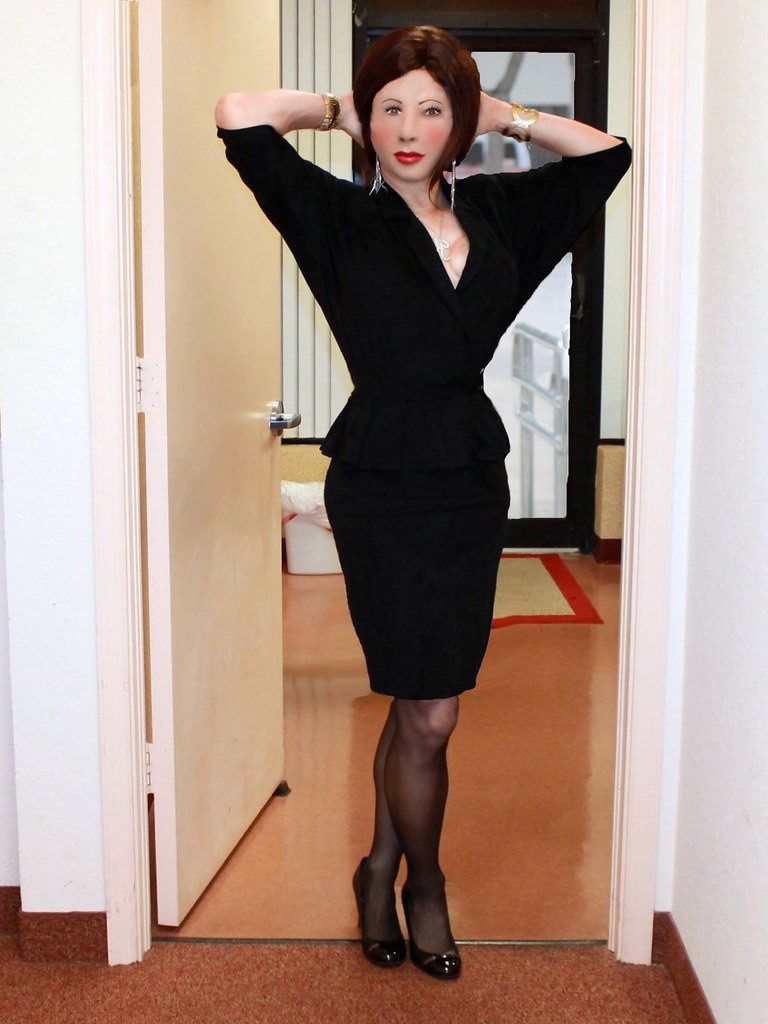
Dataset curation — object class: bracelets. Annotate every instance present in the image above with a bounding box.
[500,102,539,143]
[317,92,341,131]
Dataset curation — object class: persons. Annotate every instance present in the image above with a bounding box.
[212,23,634,980]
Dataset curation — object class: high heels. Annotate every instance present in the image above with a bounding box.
[401,881,462,980]
[352,856,407,968]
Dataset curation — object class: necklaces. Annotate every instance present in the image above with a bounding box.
[411,188,451,261]
